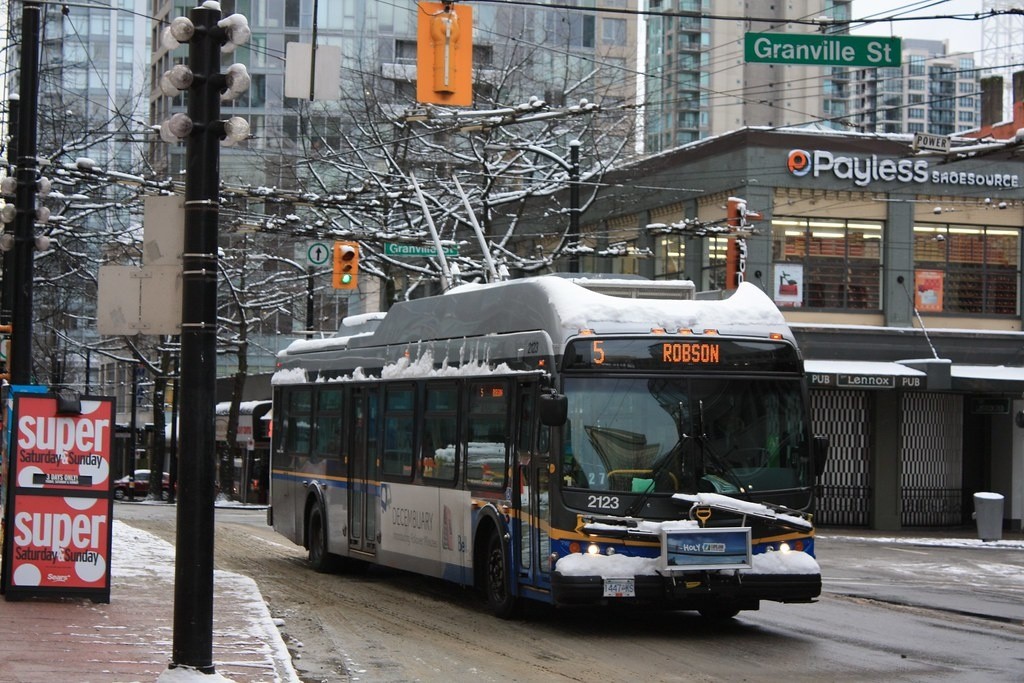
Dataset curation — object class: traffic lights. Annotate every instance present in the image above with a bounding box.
[332,241,359,290]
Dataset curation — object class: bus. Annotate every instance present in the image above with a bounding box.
[266,272,829,626]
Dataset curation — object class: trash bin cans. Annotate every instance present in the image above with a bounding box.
[973,492,1004,540]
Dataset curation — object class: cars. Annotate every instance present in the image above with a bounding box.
[112,468,177,502]
